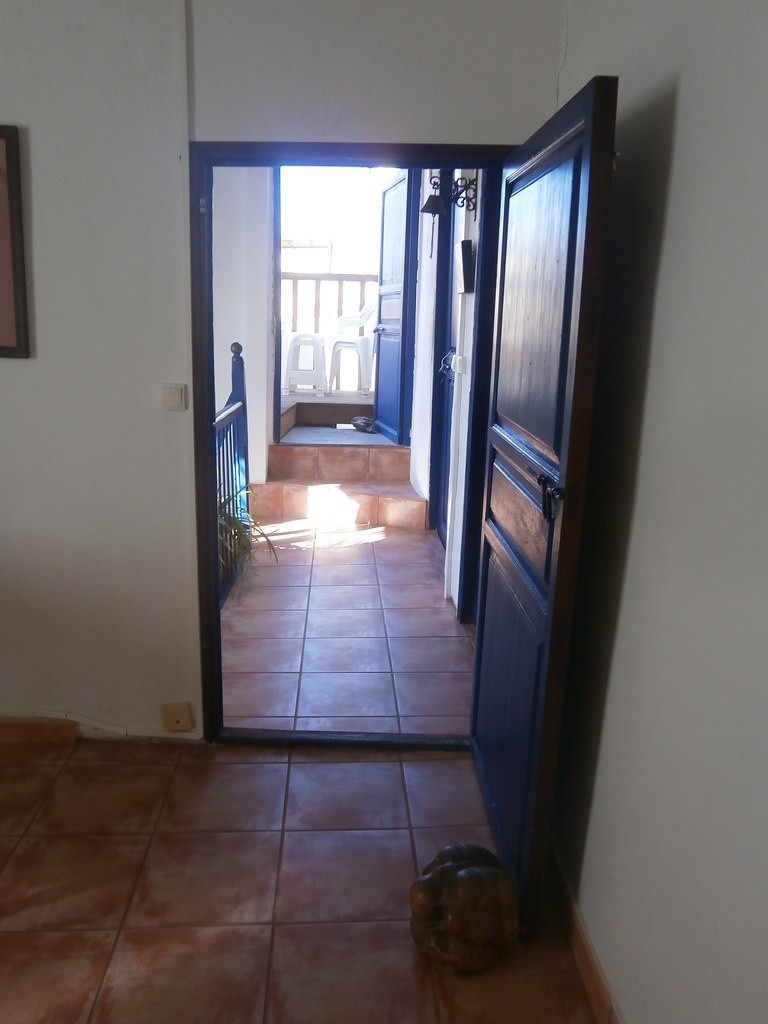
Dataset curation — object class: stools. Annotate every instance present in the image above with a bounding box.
[324,333,372,399]
[282,334,326,397]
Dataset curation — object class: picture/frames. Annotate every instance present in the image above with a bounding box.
[1,123,31,360]
